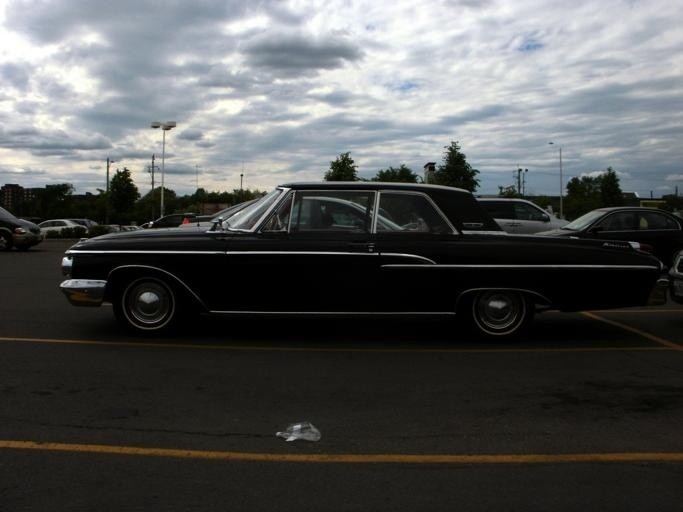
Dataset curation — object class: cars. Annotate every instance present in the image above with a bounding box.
[59,177,667,346]
[28,212,205,237]
[173,196,407,231]
[474,196,568,235]
[669,249,683,307]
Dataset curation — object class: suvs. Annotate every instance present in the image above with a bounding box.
[524,203,683,270]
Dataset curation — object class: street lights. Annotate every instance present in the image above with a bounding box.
[548,140,564,218]
[152,119,176,220]
[240,174,243,200]
[522,168,528,198]
[106,157,115,193]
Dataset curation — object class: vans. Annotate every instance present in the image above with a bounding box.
[0,206,44,254]
